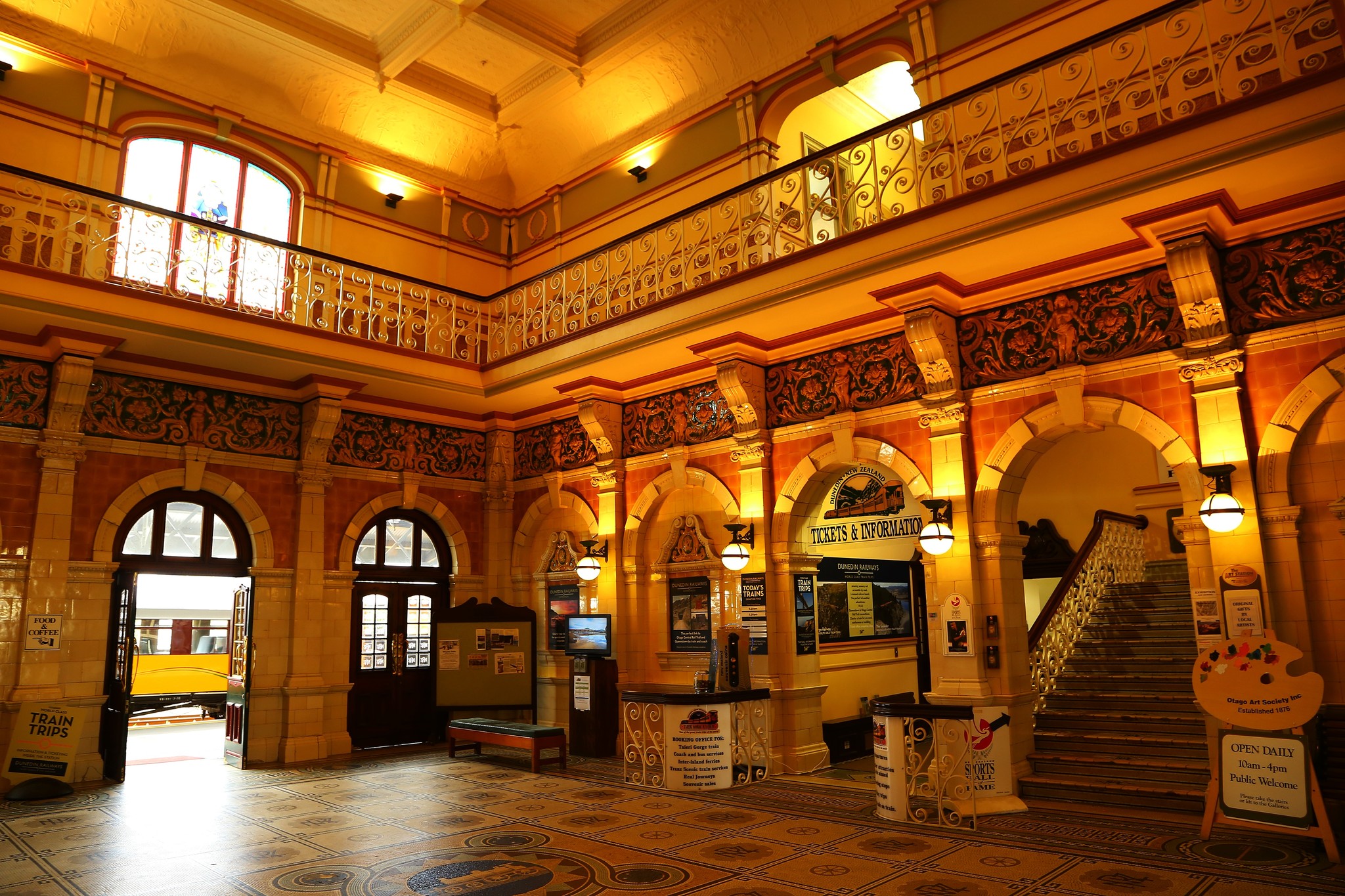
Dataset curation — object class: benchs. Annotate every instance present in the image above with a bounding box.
[449,717,569,774]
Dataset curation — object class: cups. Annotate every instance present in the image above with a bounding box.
[694,670,712,691]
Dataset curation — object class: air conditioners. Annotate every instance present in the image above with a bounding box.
[627,164,648,184]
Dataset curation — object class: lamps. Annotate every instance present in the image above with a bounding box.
[575,540,609,580]
[0,60,13,82]
[720,523,757,571]
[917,499,957,555]
[383,193,404,208]
[1196,464,1246,533]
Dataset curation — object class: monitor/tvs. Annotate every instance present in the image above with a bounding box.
[565,614,611,657]
